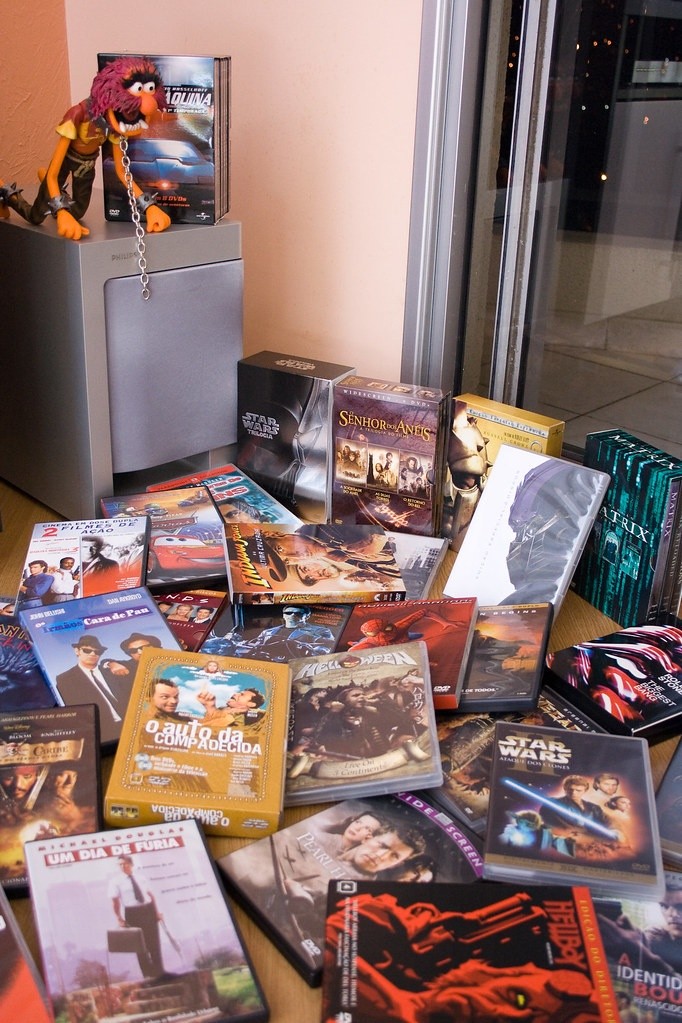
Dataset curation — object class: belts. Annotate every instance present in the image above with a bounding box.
[21,597,41,602]
[57,592,73,595]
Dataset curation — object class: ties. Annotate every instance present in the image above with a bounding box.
[90,669,124,721]
[129,875,145,903]
[192,619,197,624]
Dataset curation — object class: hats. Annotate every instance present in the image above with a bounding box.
[285,557,304,583]
[239,532,288,582]
[71,635,108,650]
[120,633,161,657]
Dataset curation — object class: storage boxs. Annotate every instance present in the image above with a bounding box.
[539,614,682,748]
[104,644,293,838]
[97,51,233,226]
[237,349,682,630]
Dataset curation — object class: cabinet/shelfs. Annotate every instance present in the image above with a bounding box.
[1,182,244,521]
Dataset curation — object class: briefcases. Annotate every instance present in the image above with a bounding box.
[106,920,148,954]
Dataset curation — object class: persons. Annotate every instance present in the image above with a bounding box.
[253,524,403,588]
[335,445,426,499]
[82,534,146,579]
[326,893,599,1023]
[277,813,436,938]
[214,498,260,524]
[16,560,54,615]
[252,605,335,664]
[50,557,79,604]
[286,668,426,759]
[345,608,465,667]
[0,757,81,822]
[111,854,165,980]
[188,606,214,624]
[501,773,632,859]
[144,660,266,728]
[0,602,15,617]
[167,604,194,621]
[157,601,173,619]
[56,633,163,733]
[597,884,682,988]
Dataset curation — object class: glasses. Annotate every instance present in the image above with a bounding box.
[282,611,305,618]
[126,643,152,654]
[78,648,104,655]
